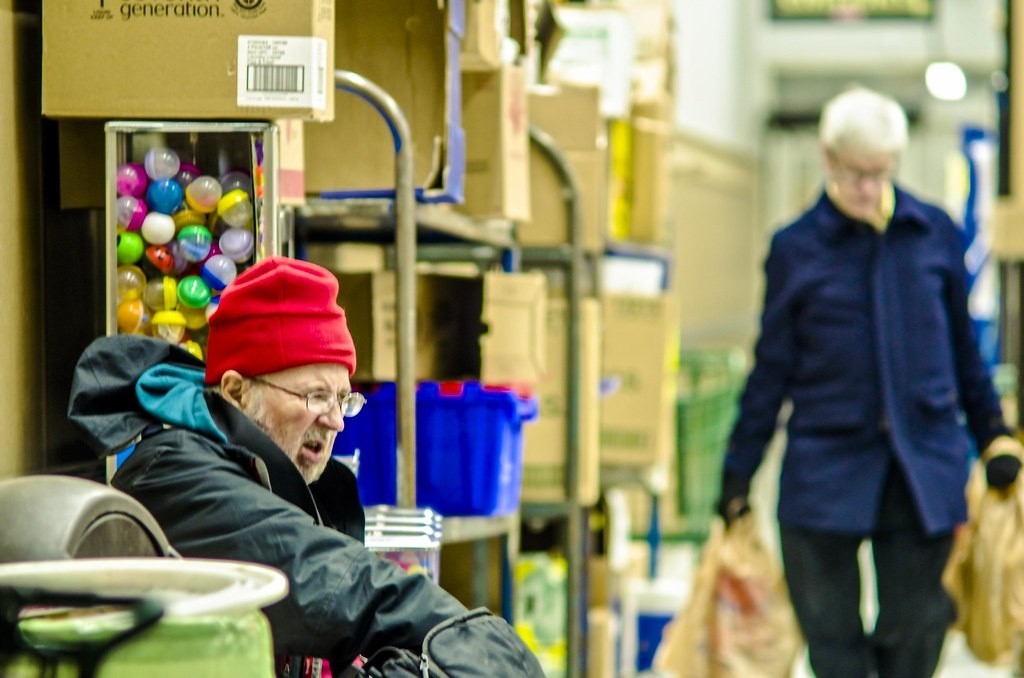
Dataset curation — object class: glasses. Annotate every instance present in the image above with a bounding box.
[249,376,367,417]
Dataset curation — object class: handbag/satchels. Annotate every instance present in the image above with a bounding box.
[367,606,547,678]
[652,515,806,678]
[940,435,1024,664]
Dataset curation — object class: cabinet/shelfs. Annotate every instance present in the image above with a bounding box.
[268,75,598,678]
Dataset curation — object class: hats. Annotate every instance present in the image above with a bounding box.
[205,256,356,385]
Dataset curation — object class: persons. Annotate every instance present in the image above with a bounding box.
[718,90,1024,678]
[110,256,547,678]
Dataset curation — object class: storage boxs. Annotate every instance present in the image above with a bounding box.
[37,0,678,505]
